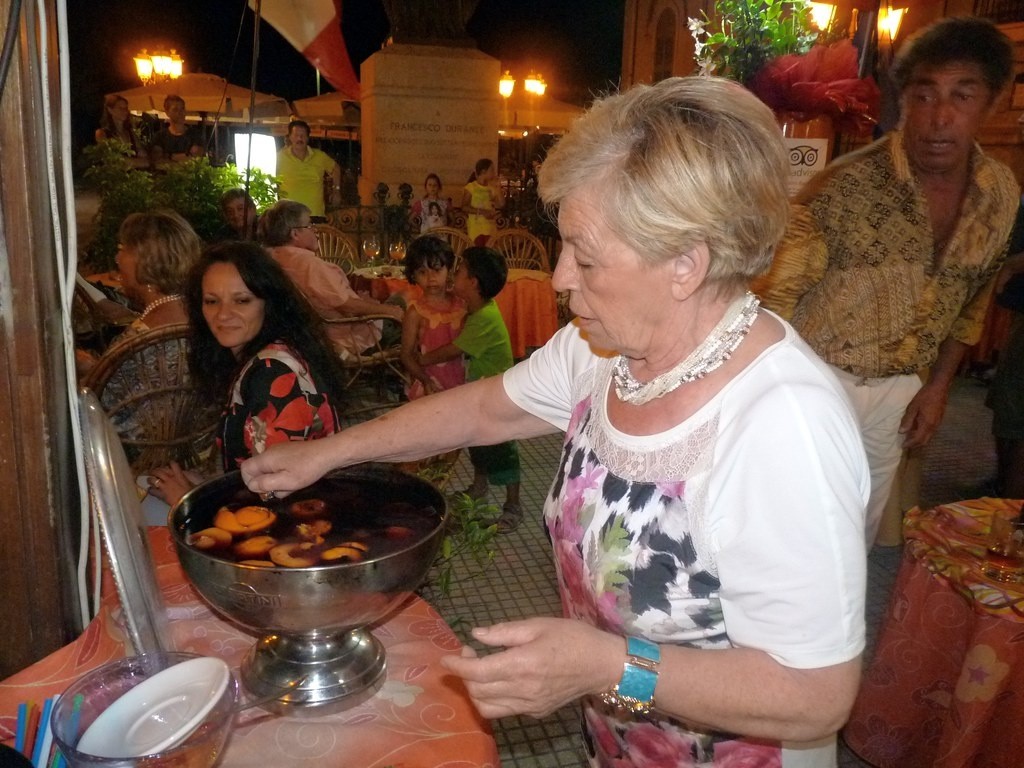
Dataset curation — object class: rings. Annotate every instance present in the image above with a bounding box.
[155,479,160,487]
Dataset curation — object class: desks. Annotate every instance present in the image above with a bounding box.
[0,463,499,768]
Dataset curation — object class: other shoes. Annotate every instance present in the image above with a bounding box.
[959,478,1001,499]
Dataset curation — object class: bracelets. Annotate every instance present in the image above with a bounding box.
[595,634,661,715]
[332,186,340,190]
[477,208,480,214]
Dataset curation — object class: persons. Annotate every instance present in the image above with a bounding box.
[145,95,204,174]
[147,240,343,510]
[95,95,150,176]
[240,76,871,768]
[400,236,469,487]
[462,158,506,245]
[276,121,341,224]
[222,189,414,403]
[407,174,455,243]
[415,246,526,534]
[984,198,1024,500]
[758,15,1021,554]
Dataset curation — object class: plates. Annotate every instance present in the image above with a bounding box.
[76,656,229,756]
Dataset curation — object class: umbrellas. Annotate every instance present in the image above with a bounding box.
[499,81,588,177]
[271,91,360,173]
[106,69,291,163]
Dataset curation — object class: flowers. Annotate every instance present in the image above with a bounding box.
[686,0,820,86]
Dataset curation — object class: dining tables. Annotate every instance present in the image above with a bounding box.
[347,264,557,359]
[839,498,1024,768]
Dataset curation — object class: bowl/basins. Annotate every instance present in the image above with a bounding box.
[50,651,240,768]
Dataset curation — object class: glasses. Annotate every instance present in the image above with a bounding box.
[288,223,315,238]
[115,239,125,250]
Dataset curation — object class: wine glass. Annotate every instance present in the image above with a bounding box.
[390,241,407,276]
[363,239,380,274]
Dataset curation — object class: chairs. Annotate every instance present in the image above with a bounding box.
[83,220,553,481]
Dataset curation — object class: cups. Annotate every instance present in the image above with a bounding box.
[981,508,1024,586]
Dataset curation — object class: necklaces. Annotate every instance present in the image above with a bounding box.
[612,290,762,405]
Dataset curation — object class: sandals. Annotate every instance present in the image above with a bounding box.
[496,503,525,534]
[461,483,488,501]
